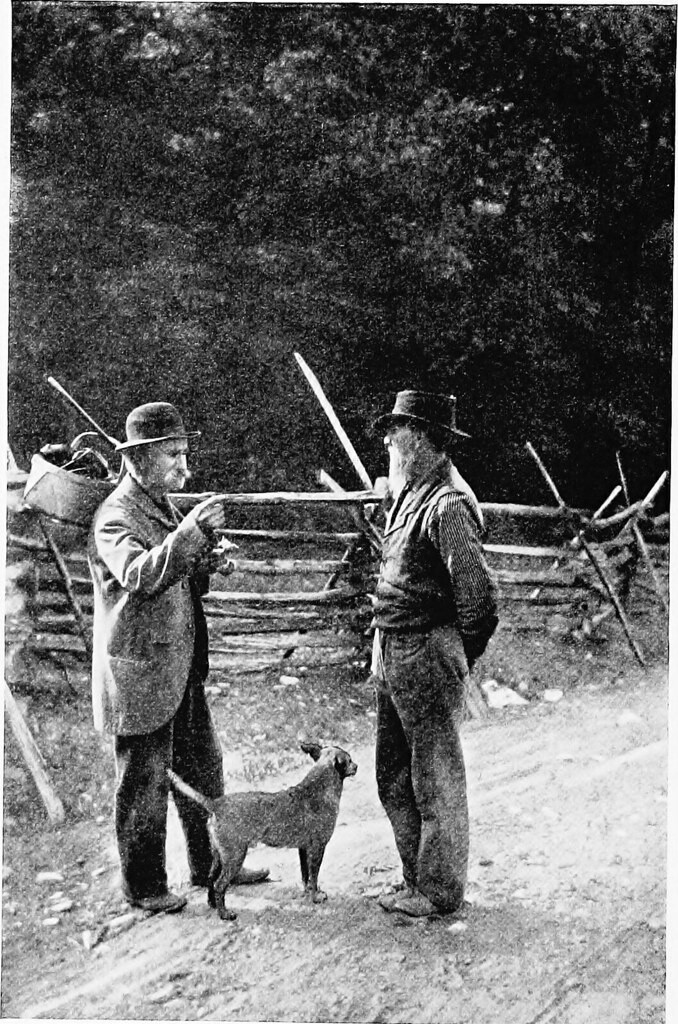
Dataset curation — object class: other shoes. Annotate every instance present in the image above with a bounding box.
[121,890,189,914]
[189,865,270,886]
[394,891,464,918]
[379,879,418,911]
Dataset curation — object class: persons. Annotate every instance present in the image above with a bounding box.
[364,388,498,914]
[86,402,270,913]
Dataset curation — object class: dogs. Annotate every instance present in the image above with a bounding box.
[166,741,359,921]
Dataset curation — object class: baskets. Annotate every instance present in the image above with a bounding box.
[24,432,128,529]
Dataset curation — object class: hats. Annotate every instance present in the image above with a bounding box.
[371,389,471,440]
[115,401,202,453]
